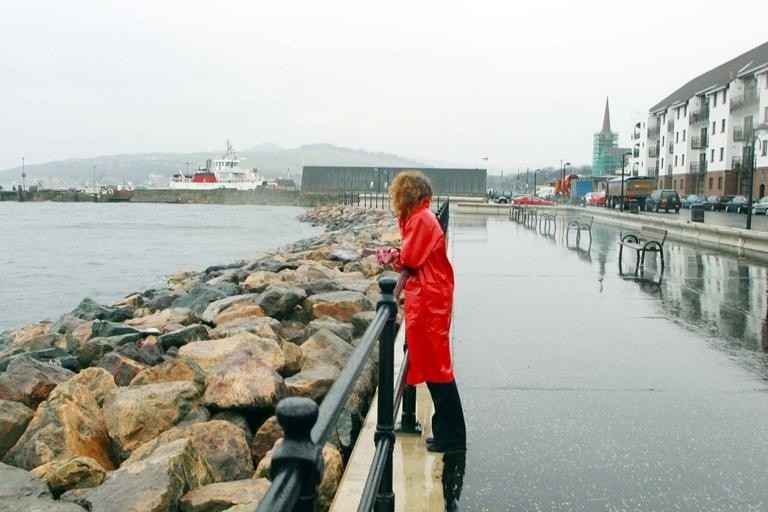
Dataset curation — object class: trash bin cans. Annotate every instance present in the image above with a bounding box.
[691,203,704,222]
[629,199,638,213]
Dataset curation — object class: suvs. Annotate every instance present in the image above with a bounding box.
[487,188,514,204]
[644,188,682,214]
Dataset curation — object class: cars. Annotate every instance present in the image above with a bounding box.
[510,194,554,206]
[678,192,767,217]
[581,191,605,207]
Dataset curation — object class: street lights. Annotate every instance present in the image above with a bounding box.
[184,159,192,192]
[525,166,530,194]
[734,161,741,194]
[374,165,380,194]
[534,167,541,197]
[516,167,520,193]
[632,162,640,176]
[91,162,97,193]
[22,156,26,191]
[620,151,633,212]
[563,162,572,200]
[745,120,767,229]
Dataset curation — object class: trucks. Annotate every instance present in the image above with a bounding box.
[603,175,658,210]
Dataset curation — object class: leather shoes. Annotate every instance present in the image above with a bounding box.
[425,438,467,453]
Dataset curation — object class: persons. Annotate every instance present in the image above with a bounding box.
[386,171,466,452]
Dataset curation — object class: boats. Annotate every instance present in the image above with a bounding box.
[168,138,264,190]
[98,183,138,204]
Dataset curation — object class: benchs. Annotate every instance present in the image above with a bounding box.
[509,203,539,230]
[617,223,668,277]
[539,209,557,237]
[565,214,595,250]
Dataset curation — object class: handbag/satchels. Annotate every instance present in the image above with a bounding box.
[376,248,402,269]
